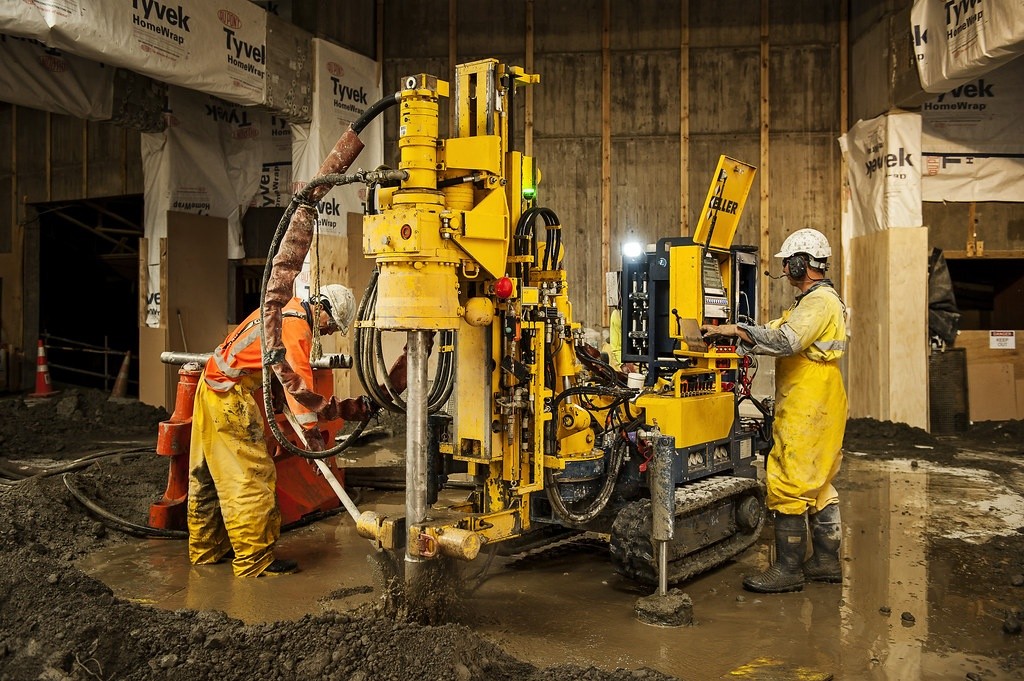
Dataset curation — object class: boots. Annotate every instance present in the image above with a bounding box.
[745,512,804,592]
[801,504,844,581]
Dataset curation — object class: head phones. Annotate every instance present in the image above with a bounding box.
[789,253,808,281]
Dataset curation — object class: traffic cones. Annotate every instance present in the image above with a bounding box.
[106,350,135,401]
[28,338,62,401]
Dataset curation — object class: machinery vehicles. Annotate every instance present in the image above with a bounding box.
[259,55,782,630]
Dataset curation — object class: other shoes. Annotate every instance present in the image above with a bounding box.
[220,552,235,564]
[264,559,300,578]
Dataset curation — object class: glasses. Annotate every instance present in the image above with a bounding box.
[781,260,790,268]
[327,318,338,333]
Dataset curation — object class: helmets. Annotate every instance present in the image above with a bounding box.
[774,228,832,259]
[318,285,356,335]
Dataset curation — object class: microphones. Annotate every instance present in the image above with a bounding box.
[764,271,789,279]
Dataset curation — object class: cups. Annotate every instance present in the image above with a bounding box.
[627,372,645,390]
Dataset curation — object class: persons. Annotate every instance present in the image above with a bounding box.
[699,228,848,591]
[186,283,356,578]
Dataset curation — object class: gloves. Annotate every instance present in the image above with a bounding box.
[701,322,737,337]
[304,431,331,476]
